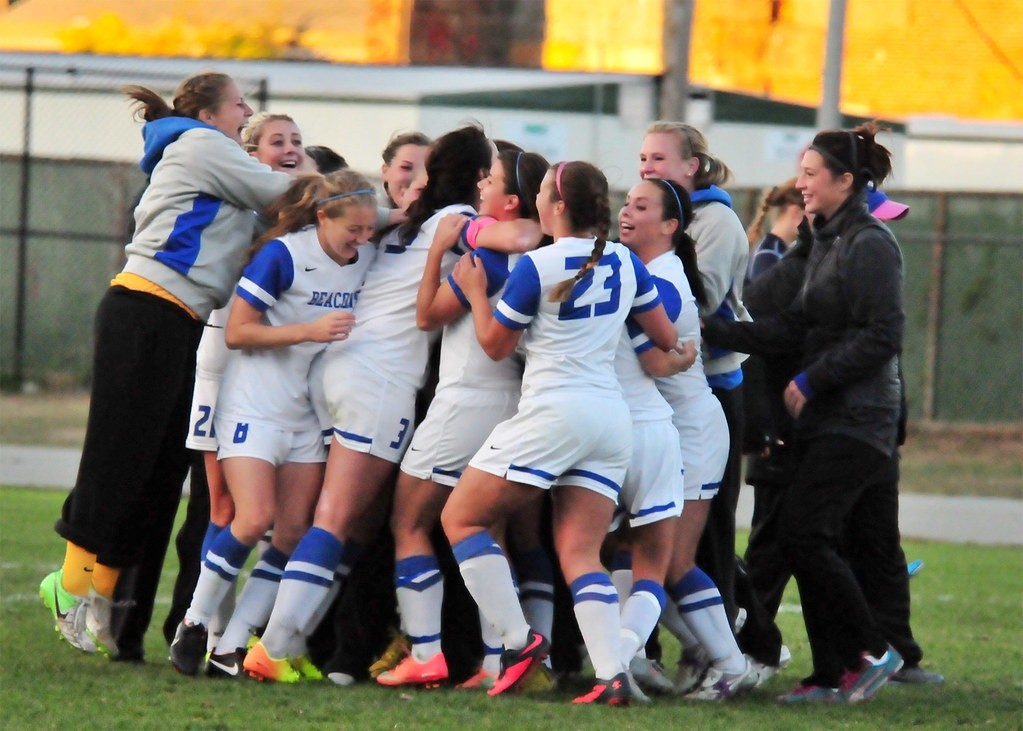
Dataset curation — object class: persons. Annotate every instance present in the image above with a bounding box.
[38,73,944,708]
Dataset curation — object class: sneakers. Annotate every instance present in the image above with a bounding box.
[843,642,904,703]
[774,682,840,703]
[630,656,675,695]
[39,569,98,653]
[205,648,247,676]
[684,656,759,702]
[170,618,208,675]
[85,581,136,658]
[247,635,324,680]
[752,645,791,687]
[369,636,411,678]
[243,642,304,682]
[455,668,500,689]
[671,642,709,695]
[487,629,551,696]
[570,673,630,705]
[624,670,652,703]
[378,642,449,688]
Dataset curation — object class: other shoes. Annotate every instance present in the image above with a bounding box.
[887,667,944,685]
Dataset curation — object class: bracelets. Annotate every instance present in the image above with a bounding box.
[670,339,686,359]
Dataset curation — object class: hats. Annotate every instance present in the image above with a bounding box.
[867,185,909,221]
